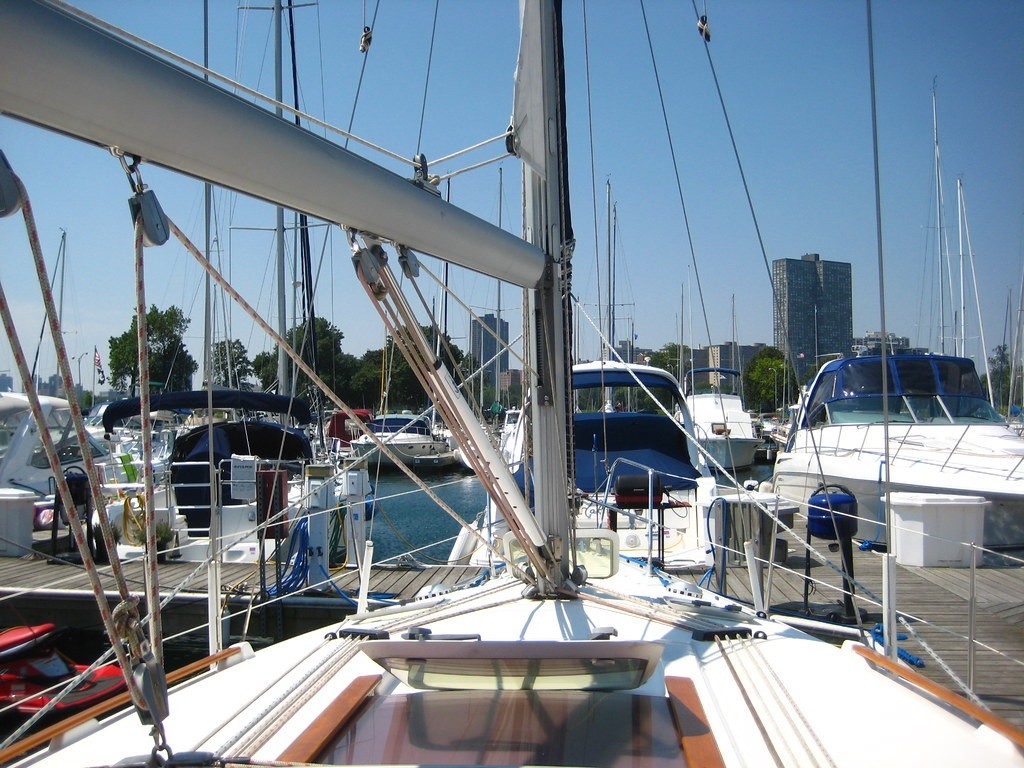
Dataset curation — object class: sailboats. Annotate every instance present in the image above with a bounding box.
[2,1,1023,768]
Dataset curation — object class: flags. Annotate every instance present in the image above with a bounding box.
[94,345,105,385]
